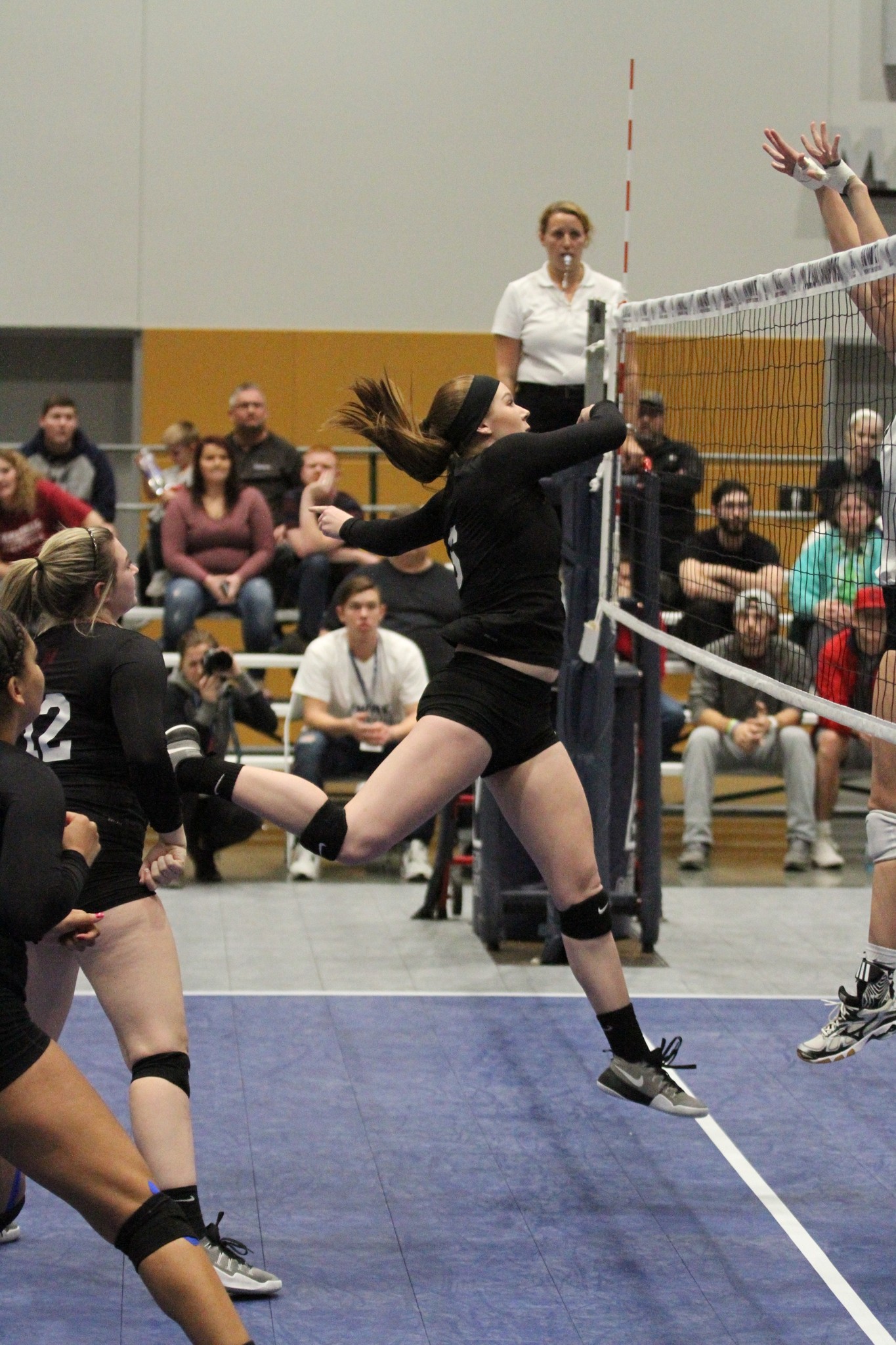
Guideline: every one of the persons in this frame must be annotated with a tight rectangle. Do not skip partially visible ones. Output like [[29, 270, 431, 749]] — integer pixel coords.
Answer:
[[0, 120, 896, 1345]]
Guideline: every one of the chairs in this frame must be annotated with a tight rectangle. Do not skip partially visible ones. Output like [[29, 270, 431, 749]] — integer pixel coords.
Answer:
[[283, 691, 391, 872]]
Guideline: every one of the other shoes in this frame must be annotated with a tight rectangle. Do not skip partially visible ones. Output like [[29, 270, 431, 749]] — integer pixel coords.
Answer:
[[678, 842, 711, 869], [811, 835, 845, 869], [782, 839, 810, 872], [185, 834, 224, 884]]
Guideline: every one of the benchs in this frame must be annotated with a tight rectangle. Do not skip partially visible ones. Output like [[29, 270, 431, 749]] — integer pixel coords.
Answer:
[[0, 441, 871, 820]]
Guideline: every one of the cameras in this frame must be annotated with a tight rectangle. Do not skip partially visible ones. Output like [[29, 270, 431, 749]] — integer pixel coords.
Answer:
[[201, 650, 233, 676]]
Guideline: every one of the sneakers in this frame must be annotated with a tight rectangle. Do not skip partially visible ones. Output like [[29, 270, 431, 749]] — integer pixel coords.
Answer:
[[198, 1210, 283, 1296], [796, 985, 896, 1064], [289, 848, 320, 881], [399, 838, 433, 880], [165, 722, 203, 771], [596, 1036, 709, 1118], [0, 1221, 21, 1242]]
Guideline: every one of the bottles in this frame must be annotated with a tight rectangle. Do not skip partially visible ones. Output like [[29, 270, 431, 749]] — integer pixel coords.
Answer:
[[138, 447, 165, 492]]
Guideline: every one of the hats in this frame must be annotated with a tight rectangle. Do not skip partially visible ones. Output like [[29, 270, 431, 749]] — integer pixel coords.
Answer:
[[734, 588, 777, 620], [637, 396, 664, 418], [712, 480, 754, 509], [854, 587, 886, 611]]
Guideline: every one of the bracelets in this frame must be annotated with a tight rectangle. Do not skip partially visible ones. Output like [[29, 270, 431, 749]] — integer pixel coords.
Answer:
[[725, 718, 738, 734], [730, 721, 741, 737]]
